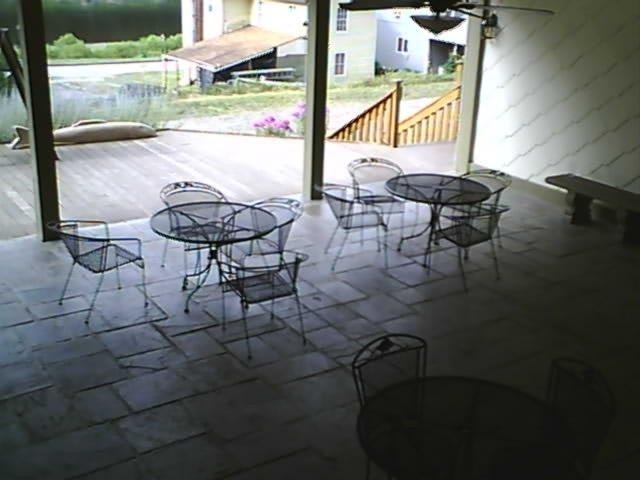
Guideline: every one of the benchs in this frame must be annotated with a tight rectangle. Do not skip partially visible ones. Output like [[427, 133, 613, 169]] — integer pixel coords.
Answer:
[[545, 172, 640, 240]]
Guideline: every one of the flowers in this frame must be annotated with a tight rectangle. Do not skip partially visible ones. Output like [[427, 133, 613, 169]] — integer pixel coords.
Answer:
[[252, 102, 307, 138]]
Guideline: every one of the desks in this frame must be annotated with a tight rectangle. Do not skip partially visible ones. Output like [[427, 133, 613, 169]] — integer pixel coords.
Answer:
[[357, 375, 577, 478]]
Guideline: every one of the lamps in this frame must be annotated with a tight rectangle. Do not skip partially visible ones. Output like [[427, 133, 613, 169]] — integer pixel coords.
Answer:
[[410, 12, 466, 36], [480, 11, 504, 39]]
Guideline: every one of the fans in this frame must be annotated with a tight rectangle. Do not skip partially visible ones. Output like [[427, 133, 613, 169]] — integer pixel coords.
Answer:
[[336, 0, 554, 24]]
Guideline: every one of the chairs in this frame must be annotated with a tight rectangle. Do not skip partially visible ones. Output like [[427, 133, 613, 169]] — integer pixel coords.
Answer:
[[350, 335, 428, 475], [347, 157, 409, 247], [218, 249, 309, 360], [314, 182, 389, 270], [435, 168, 512, 261], [546, 358, 617, 465], [221, 197, 304, 307], [160, 181, 236, 287], [428, 202, 511, 292], [45, 219, 149, 324]]
